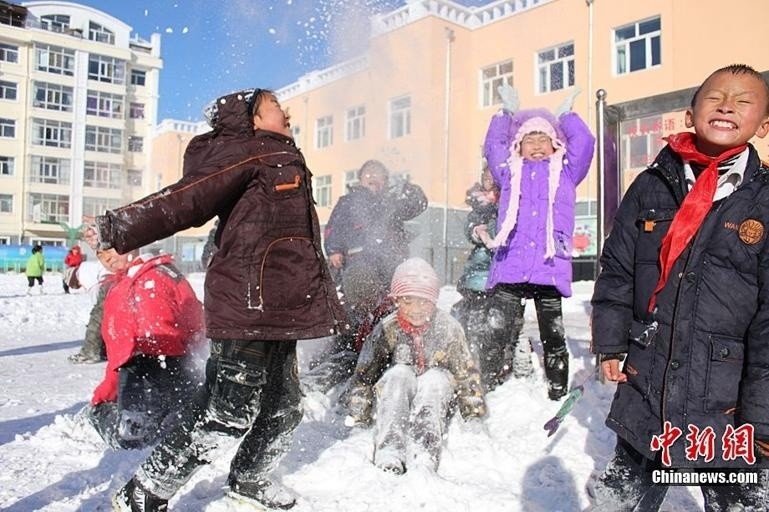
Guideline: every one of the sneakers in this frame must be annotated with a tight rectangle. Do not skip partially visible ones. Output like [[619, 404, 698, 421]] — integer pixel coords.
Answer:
[[112, 475, 168, 512], [225, 472, 296, 510]]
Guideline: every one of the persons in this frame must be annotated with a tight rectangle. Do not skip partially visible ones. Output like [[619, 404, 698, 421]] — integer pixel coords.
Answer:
[[64, 91, 594, 512], [592, 66, 769, 512], [26, 245, 45, 296]]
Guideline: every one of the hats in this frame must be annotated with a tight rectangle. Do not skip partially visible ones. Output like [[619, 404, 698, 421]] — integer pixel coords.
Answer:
[[509, 117, 564, 153], [388, 257, 439, 305]]
